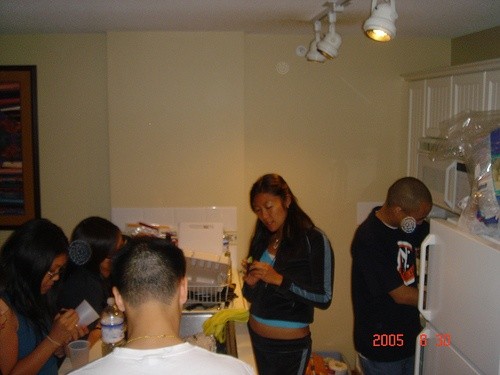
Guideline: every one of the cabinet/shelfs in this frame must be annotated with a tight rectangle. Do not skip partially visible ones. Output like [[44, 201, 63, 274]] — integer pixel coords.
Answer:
[[406, 69, 500, 178]]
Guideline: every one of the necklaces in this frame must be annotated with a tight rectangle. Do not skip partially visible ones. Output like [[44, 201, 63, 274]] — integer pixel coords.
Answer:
[[273, 240, 280, 249]]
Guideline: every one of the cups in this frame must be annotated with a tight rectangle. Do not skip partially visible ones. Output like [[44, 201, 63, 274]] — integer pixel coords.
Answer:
[[67, 340, 89, 370], [73, 299, 100, 326]]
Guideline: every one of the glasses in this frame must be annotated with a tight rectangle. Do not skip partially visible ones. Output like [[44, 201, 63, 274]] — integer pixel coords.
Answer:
[[47, 271, 60, 281]]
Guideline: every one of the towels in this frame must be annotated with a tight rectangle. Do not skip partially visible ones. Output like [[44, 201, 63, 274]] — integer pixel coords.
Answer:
[[200, 307, 251, 344]]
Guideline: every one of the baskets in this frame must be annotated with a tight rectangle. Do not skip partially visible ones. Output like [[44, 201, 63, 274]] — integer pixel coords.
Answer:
[[182, 284, 231, 314]]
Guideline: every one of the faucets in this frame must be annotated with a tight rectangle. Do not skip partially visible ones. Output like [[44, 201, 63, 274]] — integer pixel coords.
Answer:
[[222, 234, 228, 240]]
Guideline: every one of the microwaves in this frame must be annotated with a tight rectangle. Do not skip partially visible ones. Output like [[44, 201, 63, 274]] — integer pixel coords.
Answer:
[[416, 152, 469, 215]]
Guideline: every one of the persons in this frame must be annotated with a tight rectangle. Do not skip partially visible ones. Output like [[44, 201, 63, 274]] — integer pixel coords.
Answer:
[[240, 174, 335, 375], [62, 216, 122, 339], [351, 177, 433, 375], [0, 217, 88, 375], [65, 235, 255, 375]]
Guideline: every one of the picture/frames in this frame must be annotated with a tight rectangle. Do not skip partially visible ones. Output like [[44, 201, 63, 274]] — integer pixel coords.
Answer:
[[0, 64, 42, 231]]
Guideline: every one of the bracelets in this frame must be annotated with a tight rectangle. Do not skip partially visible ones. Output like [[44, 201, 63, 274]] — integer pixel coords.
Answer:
[[47, 336, 60, 345]]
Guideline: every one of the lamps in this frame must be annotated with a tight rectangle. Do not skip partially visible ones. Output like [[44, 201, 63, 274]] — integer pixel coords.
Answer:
[[306, 19, 326, 63], [317, 11, 342, 61], [364, 0, 398, 43]]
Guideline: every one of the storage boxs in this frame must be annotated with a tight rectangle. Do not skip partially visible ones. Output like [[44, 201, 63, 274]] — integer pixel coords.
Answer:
[[311, 351, 352, 375], [178, 222, 231, 295]]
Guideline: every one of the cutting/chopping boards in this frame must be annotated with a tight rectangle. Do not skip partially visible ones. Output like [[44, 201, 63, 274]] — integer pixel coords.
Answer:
[[177, 221, 223, 257]]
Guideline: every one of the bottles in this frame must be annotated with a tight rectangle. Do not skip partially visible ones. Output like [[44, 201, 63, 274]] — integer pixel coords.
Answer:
[[101, 297, 124, 356]]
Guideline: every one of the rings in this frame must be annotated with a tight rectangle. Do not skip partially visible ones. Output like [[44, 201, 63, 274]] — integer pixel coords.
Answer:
[[74, 324, 77, 327]]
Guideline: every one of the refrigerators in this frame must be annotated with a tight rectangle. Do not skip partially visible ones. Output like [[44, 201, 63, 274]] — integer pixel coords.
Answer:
[[412, 217, 500, 375]]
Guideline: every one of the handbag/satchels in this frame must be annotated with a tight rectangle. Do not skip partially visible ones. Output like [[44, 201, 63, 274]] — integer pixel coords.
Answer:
[[305, 352, 331, 375]]
